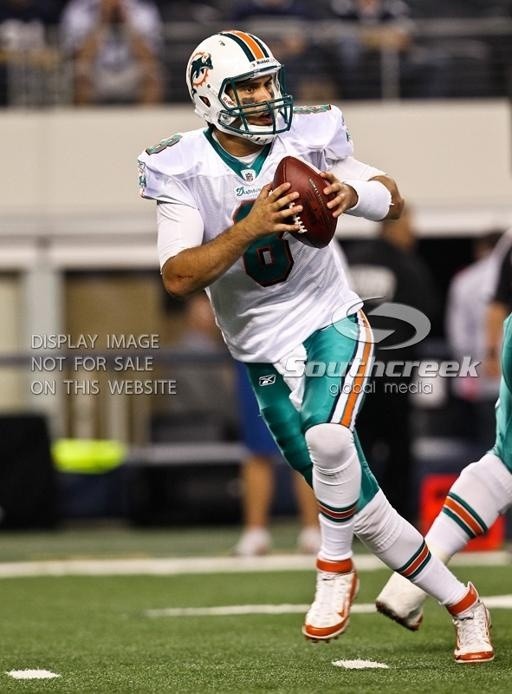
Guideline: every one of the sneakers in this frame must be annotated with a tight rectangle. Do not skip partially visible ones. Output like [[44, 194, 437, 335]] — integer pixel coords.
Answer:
[[374, 574, 424, 630], [448, 582, 496, 664], [301, 559, 360, 645]]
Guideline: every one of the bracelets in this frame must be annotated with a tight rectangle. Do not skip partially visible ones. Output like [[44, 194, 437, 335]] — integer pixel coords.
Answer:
[[339, 177, 394, 223]]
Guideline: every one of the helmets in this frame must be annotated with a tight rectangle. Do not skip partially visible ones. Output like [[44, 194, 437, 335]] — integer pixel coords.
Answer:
[[185, 29, 295, 148]]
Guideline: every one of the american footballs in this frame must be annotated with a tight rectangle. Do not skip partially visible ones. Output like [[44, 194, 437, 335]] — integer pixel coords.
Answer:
[[272, 156, 337, 249]]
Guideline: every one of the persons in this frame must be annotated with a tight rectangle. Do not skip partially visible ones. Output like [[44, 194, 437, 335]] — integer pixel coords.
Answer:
[[346, 199, 446, 529], [229, 359, 321, 560], [3, 1, 512, 107], [136, 26, 498, 665], [371, 311, 512, 634], [448, 234, 507, 460]]
